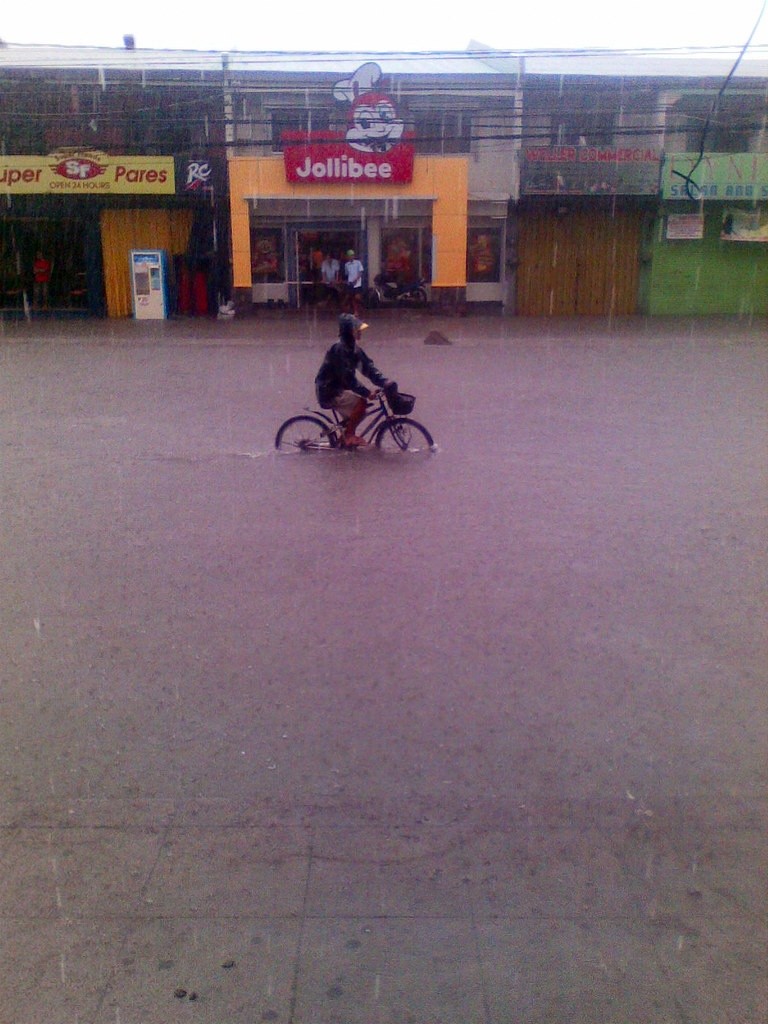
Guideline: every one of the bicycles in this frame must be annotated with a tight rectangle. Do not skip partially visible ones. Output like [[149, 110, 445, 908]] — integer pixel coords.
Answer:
[[275, 383, 434, 452]]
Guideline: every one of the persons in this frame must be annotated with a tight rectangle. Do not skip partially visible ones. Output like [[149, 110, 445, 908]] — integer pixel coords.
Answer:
[[318, 253, 341, 309], [315, 313, 397, 452], [342, 250, 365, 317], [33, 248, 51, 313]]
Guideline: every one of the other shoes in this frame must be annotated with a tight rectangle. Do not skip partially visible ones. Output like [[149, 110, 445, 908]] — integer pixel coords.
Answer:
[[345, 436, 368, 446], [42, 305, 48, 311], [32, 303, 38, 311]]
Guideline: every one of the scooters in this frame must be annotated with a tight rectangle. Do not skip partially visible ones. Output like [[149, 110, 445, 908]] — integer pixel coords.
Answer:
[[370, 269, 427, 307]]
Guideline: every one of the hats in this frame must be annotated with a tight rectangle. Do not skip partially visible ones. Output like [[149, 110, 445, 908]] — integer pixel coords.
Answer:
[[347, 250, 354, 257], [352, 316, 369, 335]]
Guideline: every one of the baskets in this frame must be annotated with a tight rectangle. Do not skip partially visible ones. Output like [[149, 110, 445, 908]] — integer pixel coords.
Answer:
[[386, 392, 416, 415]]
